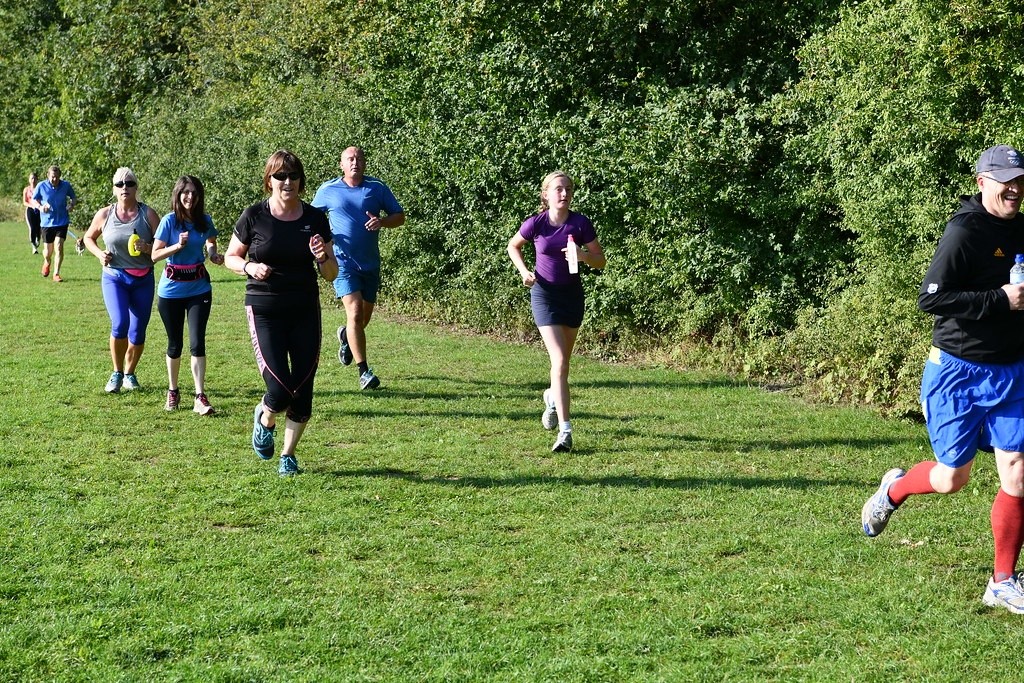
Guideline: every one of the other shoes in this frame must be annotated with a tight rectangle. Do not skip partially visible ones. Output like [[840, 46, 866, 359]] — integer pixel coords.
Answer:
[[32, 246, 38, 254]]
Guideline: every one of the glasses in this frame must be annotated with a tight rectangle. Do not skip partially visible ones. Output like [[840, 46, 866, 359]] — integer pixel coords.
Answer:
[[271, 171, 302, 181], [113, 179, 138, 188], [979, 174, 1024, 189]]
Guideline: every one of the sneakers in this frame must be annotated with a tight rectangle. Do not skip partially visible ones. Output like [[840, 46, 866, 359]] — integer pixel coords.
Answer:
[[52, 274, 64, 282], [42, 260, 51, 277], [543, 388, 559, 429], [861, 467, 907, 538], [358, 368, 381, 390], [165, 387, 181, 412], [122, 374, 140, 389], [336, 325, 353, 365], [252, 403, 278, 459], [982, 572, 1024, 616], [278, 453, 298, 477], [552, 429, 572, 452], [193, 393, 216, 415], [105, 371, 124, 393]]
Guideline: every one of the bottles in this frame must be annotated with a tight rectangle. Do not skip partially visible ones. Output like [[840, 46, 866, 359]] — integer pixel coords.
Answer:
[[1010, 255, 1024, 287], [567, 235, 578, 274], [128, 229, 141, 256]]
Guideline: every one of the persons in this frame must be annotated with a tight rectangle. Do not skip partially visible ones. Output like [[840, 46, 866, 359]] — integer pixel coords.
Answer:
[[860, 145, 1024, 615], [506, 172, 606, 452], [309, 146, 405, 390], [83, 166, 161, 393], [224, 149, 338, 478], [22, 163, 78, 282], [151, 174, 224, 416]]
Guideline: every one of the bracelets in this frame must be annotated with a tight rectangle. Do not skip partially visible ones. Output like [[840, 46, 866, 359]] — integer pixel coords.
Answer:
[[243, 261, 253, 276], [316, 253, 329, 264]]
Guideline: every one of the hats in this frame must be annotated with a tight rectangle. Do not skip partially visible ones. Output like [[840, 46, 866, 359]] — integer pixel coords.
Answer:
[[976, 145, 1024, 183]]
